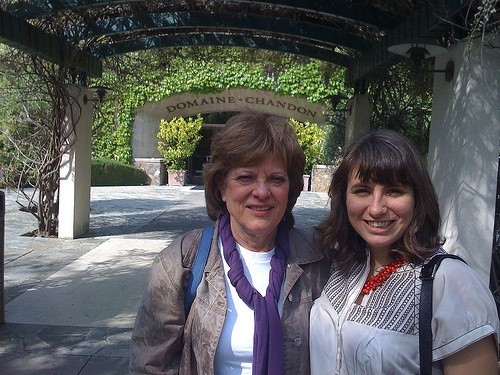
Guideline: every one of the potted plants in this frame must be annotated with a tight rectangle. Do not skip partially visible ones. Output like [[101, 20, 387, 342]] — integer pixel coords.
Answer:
[[156, 114, 203, 187], [290, 117, 330, 191]]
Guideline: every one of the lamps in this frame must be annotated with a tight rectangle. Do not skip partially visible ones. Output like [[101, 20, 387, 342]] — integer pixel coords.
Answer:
[[82, 86, 116, 105], [387, 38, 454, 82]]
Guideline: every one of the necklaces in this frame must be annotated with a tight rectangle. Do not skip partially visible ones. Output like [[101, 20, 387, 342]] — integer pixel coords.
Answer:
[[359, 255, 417, 296]]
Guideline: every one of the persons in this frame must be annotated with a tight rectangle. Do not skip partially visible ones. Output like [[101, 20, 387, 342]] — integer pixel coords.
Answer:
[[128, 110, 332, 375], [307, 124, 500, 375]]
